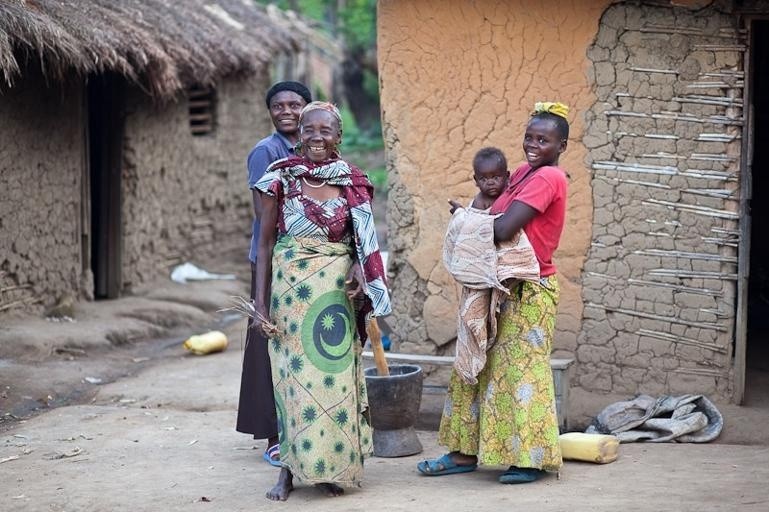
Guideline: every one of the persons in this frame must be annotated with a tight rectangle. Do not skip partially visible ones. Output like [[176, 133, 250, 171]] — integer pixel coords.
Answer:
[[417, 102, 568, 483], [472, 148, 511, 209], [252, 100, 395, 501], [236, 82, 312, 466]]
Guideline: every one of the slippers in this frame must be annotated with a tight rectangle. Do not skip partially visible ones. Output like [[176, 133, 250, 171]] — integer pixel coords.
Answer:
[[263, 444, 282, 467], [499, 467, 537, 484], [417, 454, 474, 476]]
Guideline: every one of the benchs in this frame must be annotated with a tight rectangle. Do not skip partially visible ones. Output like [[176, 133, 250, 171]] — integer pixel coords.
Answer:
[[362, 350, 575, 435]]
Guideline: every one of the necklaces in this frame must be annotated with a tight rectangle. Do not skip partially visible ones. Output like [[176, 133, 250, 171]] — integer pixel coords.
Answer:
[[302, 177, 329, 188]]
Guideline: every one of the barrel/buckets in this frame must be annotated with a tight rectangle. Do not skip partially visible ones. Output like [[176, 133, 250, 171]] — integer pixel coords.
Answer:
[[183, 331, 228, 355]]
